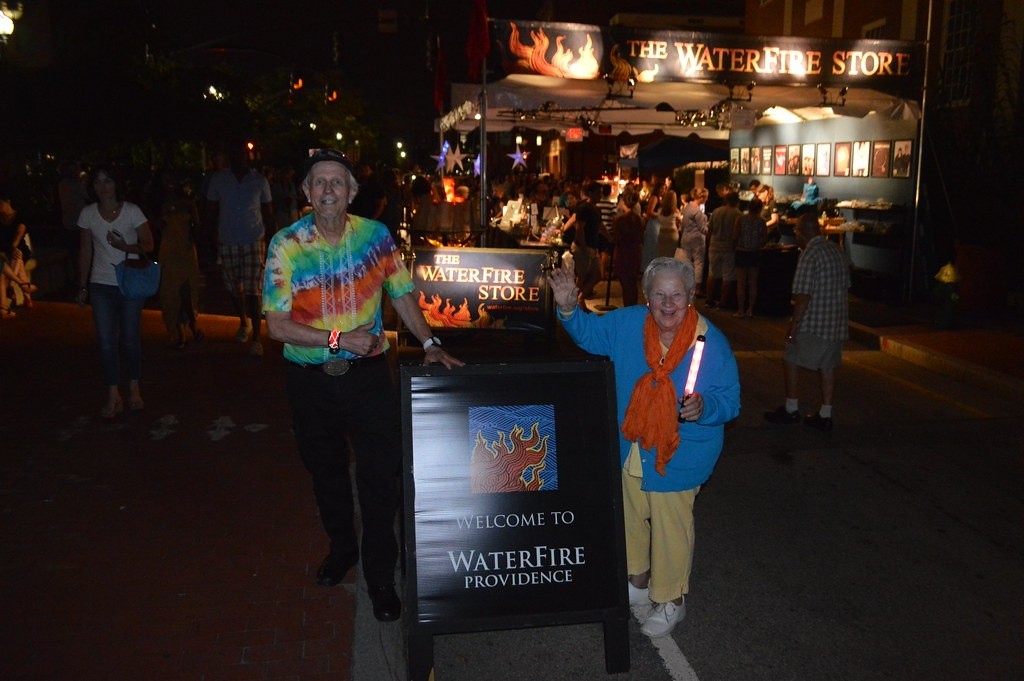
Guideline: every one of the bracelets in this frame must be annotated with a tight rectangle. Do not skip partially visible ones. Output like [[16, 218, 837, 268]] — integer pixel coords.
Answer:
[[558, 308, 574, 316], [328, 330, 341, 355]]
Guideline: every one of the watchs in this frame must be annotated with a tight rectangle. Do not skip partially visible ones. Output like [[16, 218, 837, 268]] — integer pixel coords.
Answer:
[[79, 287, 88, 292], [423, 336, 442, 352]]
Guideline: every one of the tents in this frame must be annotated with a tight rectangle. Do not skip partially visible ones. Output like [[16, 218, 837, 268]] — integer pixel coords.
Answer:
[[429, 15, 935, 248]]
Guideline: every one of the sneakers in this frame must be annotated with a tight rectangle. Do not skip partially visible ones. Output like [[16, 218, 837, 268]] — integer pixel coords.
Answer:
[[628, 581, 650, 605], [641, 594, 685, 637]]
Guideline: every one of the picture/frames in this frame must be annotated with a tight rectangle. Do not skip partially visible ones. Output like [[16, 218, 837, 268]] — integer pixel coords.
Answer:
[[730, 138, 914, 180]]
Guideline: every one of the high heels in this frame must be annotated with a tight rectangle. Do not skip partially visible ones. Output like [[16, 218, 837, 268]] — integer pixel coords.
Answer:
[[131, 398, 144, 410], [101, 400, 123, 419]]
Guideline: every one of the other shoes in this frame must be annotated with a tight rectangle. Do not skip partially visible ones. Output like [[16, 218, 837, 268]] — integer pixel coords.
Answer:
[[704, 301, 716, 307], [746, 312, 753, 319], [716, 303, 732, 310], [733, 311, 744, 318], [248, 342, 262, 356], [235, 329, 250, 343]]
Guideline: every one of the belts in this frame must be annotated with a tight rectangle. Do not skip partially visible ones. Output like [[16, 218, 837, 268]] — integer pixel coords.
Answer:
[[305, 355, 373, 376]]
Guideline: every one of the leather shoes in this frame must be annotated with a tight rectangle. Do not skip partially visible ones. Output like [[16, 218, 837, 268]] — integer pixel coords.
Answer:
[[764, 405, 801, 424], [369, 582, 399, 621], [316, 546, 359, 587], [805, 411, 833, 431]]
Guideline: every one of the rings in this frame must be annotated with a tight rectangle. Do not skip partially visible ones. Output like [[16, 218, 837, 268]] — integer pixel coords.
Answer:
[[788, 336, 792, 339], [108, 240, 112, 244]]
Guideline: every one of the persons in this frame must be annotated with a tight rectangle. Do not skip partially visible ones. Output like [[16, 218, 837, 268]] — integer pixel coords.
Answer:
[[146, 163, 209, 349], [487, 169, 779, 319], [75, 161, 156, 418], [350, 149, 481, 249], [791, 177, 823, 211], [258, 146, 467, 621], [196, 133, 305, 356], [762, 211, 851, 432], [0, 186, 38, 319], [541, 258, 740, 640]]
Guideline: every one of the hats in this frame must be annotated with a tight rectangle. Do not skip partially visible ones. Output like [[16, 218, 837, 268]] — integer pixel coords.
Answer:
[[303, 149, 352, 177]]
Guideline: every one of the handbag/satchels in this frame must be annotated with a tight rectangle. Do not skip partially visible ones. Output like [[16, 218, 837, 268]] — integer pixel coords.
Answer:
[[114, 244, 163, 299]]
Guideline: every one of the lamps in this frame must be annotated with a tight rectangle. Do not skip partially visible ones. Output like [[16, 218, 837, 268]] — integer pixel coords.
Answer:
[[722, 78, 757, 102], [601, 74, 636, 99], [815, 83, 849, 108]]
